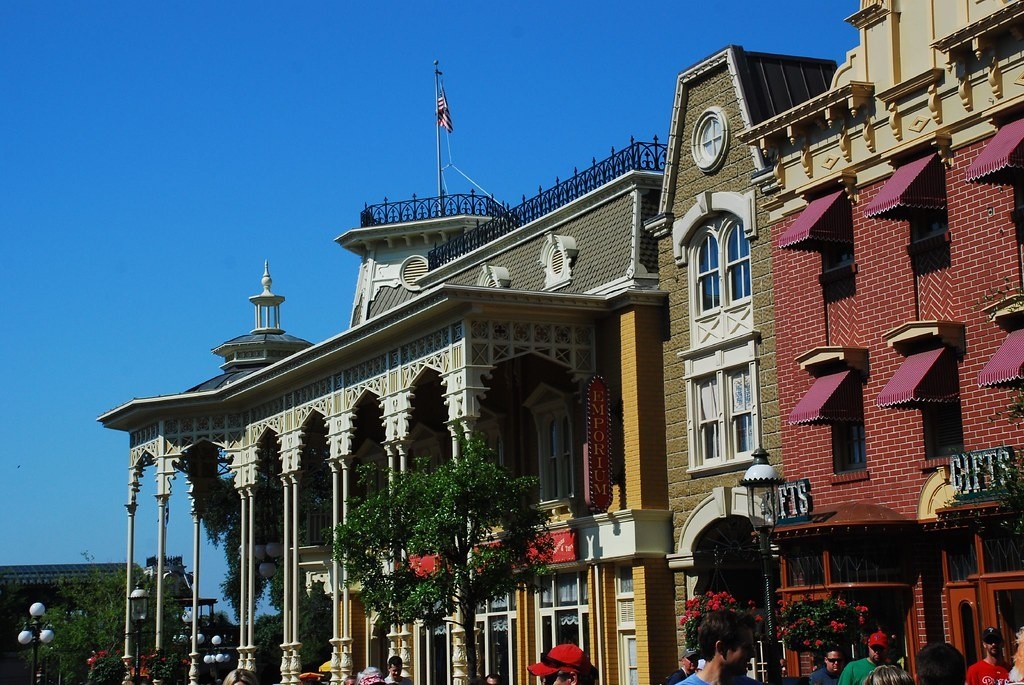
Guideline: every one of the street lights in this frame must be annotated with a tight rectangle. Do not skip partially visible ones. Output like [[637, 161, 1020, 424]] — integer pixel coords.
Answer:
[[18, 602, 54, 685], [173, 610, 205, 685], [128, 579, 151, 685], [203, 635, 231, 685], [739, 443, 787, 685]]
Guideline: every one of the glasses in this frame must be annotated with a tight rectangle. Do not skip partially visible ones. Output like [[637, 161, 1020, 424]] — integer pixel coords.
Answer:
[[872, 645, 884, 652], [828, 658, 841, 663], [985, 638, 1001, 644]]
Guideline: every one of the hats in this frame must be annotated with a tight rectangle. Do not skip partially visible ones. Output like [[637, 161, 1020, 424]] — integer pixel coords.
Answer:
[[682, 648, 700, 658], [528, 644, 587, 677], [982, 627, 1002, 641], [300, 672, 324, 682], [358, 667, 385, 685], [868, 630, 889, 648]]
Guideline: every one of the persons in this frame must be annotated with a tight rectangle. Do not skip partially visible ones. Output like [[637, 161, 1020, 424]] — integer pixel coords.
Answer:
[[221, 609, 1024, 685]]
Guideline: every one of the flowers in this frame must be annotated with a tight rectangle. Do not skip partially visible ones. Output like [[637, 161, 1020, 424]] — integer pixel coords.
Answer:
[[680, 589, 766, 652], [774, 595, 872, 651]]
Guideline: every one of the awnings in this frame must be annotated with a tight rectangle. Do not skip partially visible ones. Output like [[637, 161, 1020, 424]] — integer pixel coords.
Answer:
[[789, 370, 864, 424], [875, 347, 960, 408], [777, 190, 854, 254], [966, 118, 1024, 185], [862, 154, 946, 221], [976, 328, 1024, 390]]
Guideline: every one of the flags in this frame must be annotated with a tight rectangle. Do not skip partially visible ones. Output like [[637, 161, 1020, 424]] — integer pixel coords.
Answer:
[[435, 85, 453, 133]]
